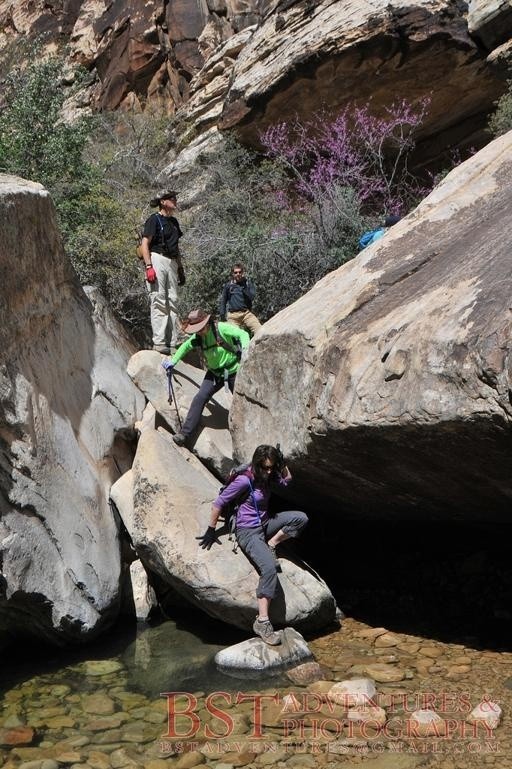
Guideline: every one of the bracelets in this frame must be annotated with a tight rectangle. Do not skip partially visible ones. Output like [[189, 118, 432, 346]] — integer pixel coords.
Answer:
[[145, 263, 152, 266]]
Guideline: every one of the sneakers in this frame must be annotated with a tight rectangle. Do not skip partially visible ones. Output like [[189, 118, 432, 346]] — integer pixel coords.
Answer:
[[266, 541, 281, 570], [152, 343, 171, 356], [252, 614, 282, 646], [172, 432, 187, 447]]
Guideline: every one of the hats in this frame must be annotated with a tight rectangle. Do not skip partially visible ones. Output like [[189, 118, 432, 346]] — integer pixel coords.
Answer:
[[148, 189, 182, 208], [184, 309, 212, 334]]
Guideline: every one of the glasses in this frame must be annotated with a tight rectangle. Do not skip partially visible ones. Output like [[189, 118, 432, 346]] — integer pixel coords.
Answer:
[[259, 463, 276, 471], [233, 271, 242, 274], [165, 196, 178, 202]]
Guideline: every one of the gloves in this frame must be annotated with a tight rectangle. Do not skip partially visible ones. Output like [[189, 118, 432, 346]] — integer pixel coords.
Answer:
[[220, 314, 228, 322], [195, 525, 223, 551], [144, 263, 158, 284], [177, 266, 186, 287], [161, 359, 175, 370]]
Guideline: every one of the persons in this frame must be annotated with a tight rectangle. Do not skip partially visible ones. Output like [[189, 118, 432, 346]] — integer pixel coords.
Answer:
[[160, 308, 251, 447], [219, 263, 262, 335], [358, 215, 401, 248], [138, 188, 187, 355], [197, 444, 309, 644]]
